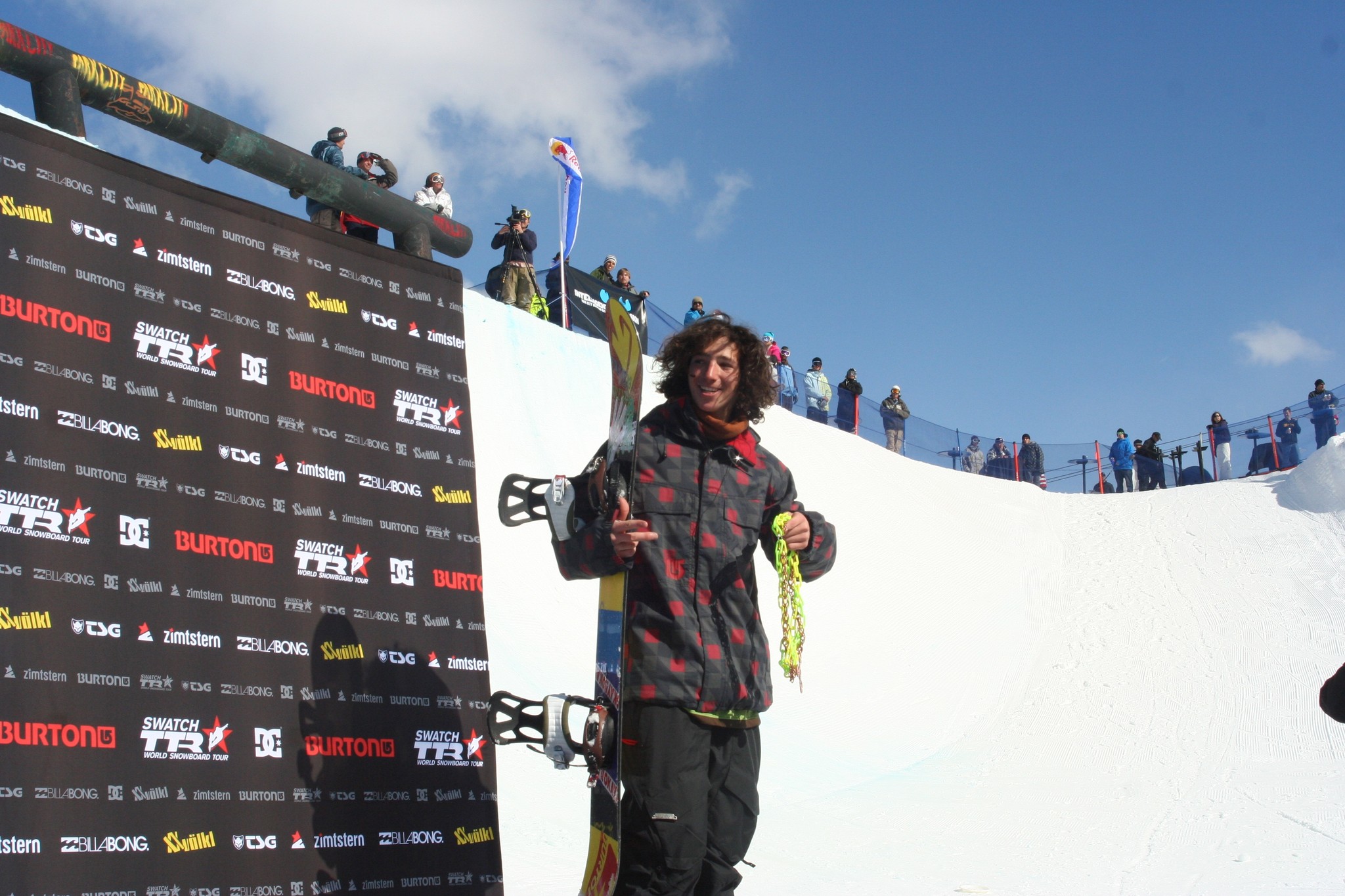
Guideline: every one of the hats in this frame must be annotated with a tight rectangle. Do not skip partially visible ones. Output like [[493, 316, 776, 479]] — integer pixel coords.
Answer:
[[692, 297, 703, 308], [812, 357, 822, 367], [327, 127, 374, 165], [848, 368, 856, 378], [763, 332, 774, 342], [1116, 428, 1162, 446], [603, 255, 617, 268], [891, 385, 900, 391], [1314, 379, 1325, 388], [551, 251, 569, 261]]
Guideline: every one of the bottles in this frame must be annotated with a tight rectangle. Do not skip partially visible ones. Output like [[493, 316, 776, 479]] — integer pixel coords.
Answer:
[[1288, 427, 1291, 434]]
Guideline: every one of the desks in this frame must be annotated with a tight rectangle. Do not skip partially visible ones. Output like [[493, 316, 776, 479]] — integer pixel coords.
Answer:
[[988, 458, 1031, 482], [1306, 408, 1342, 438], [1067, 459, 1098, 494], [1248, 442, 1282, 472], [937, 450, 971, 470], [1237, 433, 1271, 474]]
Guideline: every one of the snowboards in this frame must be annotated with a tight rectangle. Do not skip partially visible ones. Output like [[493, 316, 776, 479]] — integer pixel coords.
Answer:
[[577, 296, 643, 896]]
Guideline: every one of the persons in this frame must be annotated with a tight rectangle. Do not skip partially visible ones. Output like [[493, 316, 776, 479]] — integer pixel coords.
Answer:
[[1308, 379, 1339, 450], [987, 438, 1011, 464], [1206, 412, 1232, 481], [962, 436, 984, 475], [880, 385, 910, 454], [684, 297, 706, 329], [1276, 407, 1301, 468], [343, 151, 398, 243], [1018, 434, 1045, 487], [1141, 432, 1167, 491], [306, 127, 370, 234], [1108, 428, 1134, 493], [1132, 439, 1150, 492], [834, 368, 863, 434], [486, 209, 569, 327], [550, 312, 836, 896], [1093, 472, 1115, 493], [590, 255, 617, 285], [804, 357, 832, 425], [412, 173, 453, 219], [761, 332, 782, 382], [614, 268, 650, 297], [776, 346, 799, 412]]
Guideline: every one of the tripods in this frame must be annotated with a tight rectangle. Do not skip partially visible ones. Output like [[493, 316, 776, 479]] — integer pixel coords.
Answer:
[[495, 226, 549, 322]]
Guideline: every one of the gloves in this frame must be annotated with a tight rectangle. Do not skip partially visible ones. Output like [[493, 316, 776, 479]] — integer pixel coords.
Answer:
[[793, 396, 827, 408], [371, 152, 382, 163], [1206, 424, 1213, 429]]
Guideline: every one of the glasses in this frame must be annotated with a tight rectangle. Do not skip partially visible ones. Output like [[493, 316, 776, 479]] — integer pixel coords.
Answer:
[[328, 129, 444, 184], [813, 361, 821, 366], [847, 371, 856, 376], [519, 209, 531, 219], [1117, 432, 1124, 434], [693, 302, 701, 305], [971, 439, 980, 443], [780, 350, 790, 357], [762, 336, 773, 342], [1319, 383, 1324, 385], [891, 389, 900, 393]]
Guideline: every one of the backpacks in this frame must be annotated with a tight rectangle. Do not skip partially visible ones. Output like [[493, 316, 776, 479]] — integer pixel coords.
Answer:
[[530, 293, 548, 322]]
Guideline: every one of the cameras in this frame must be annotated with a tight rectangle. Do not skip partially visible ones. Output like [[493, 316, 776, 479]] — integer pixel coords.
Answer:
[[508, 206, 523, 227]]
